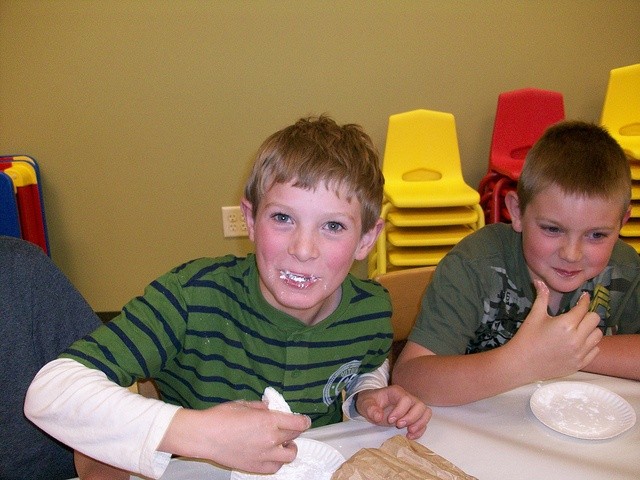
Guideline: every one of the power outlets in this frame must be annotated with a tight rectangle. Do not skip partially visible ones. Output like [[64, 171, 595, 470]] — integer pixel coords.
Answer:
[[221, 205, 249, 239]]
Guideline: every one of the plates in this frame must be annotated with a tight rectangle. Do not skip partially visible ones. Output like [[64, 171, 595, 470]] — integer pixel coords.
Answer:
[[230, 436, 346, 480], [530, 381, 636, 440]]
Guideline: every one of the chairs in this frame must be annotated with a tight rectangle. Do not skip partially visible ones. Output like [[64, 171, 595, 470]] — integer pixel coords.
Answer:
[[366, 109, 486, 278], [597, 63, 640, 255], [479, 87, 566, 226]]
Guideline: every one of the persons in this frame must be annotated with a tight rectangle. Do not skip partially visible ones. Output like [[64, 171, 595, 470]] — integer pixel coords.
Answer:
[[0, 236, 130, 480], [24, 114, 432, 480], [392, 121, 640, 407]]
